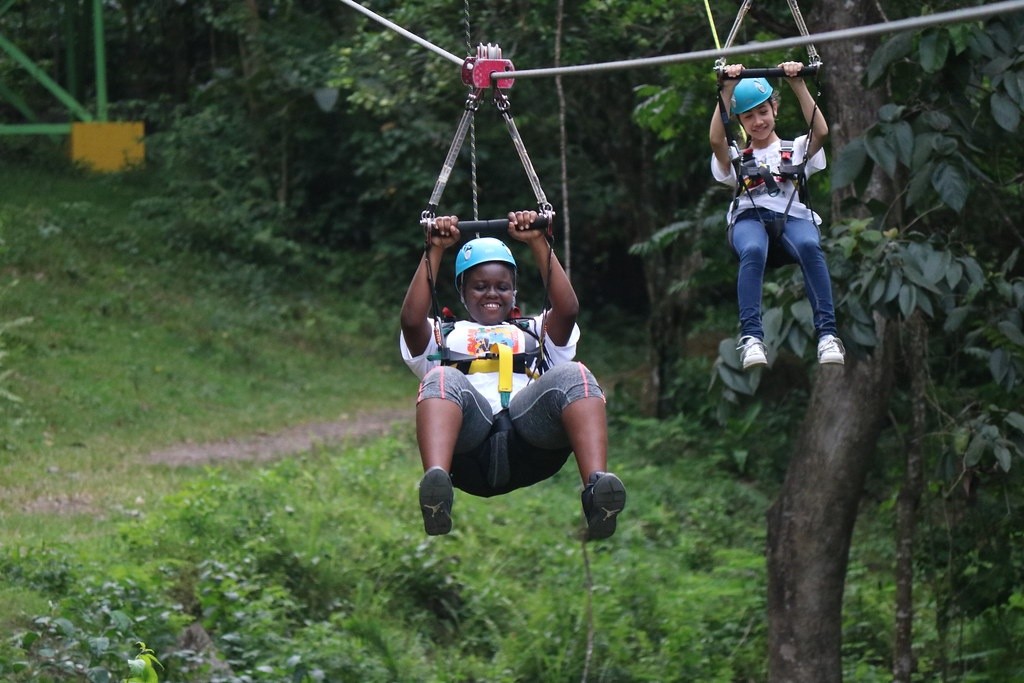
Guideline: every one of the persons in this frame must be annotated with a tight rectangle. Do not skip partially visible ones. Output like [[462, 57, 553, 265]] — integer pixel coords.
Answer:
[[399, 210, 627, 539], [708, 60, 847, 370]]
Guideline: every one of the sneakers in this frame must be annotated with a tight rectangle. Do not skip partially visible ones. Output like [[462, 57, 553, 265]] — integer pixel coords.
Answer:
[[419, 467, 453, 535], [817, 335, 846, 365], [737, 336, 769, 369], [582, 469, 626, 539]]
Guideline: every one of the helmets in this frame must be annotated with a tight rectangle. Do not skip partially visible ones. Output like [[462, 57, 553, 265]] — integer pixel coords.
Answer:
[[731, 78, 773, 114], [454, 237, 516, 292]]
